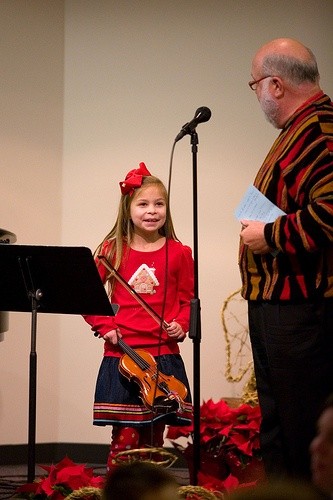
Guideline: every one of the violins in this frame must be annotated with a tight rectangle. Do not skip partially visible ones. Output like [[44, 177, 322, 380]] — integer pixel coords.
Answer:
[[94, 330, 188, 413]]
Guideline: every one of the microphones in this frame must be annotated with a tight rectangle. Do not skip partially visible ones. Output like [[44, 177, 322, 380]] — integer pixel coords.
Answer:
[[175, 106, 211, 141]]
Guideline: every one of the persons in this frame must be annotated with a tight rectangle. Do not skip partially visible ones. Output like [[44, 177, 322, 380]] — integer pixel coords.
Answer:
[[310, 404, 333, 500], [227, 475, 323, 500], [239, 38, 333, 484], [79, 175, 194, 477], [103, 461, 182, 500]]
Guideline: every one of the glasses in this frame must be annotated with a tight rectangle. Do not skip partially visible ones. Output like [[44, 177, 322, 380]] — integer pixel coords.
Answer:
[[248, 76, 274, 90]]
